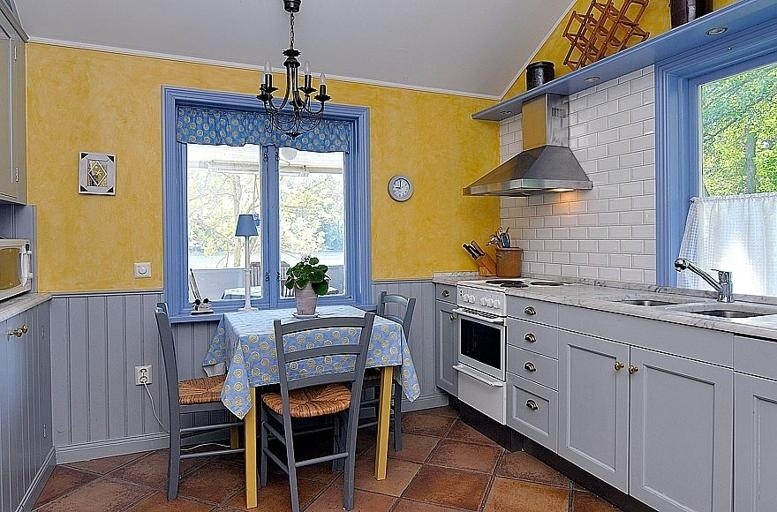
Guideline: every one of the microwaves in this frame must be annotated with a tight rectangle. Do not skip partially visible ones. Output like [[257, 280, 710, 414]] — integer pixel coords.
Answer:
[[0, 239, 34, 301]]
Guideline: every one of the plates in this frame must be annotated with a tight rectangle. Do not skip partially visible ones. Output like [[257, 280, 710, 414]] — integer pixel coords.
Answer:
[[292, 312, 318, 319]]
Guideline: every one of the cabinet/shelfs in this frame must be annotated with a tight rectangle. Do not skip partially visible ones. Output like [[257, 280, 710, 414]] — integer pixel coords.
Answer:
[[506, 295, 559, 454], [734, 332, 776, 512], [558, 310, 733, 512], [1, 300, 52, 512], [0, 9, 27, 205], [434, 285, 457, 395]]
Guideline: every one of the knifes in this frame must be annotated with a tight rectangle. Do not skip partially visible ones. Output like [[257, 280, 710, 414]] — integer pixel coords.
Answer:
[[462, 240, 484, 259]]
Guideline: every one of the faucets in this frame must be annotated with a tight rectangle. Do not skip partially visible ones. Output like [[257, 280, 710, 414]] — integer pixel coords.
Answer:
[[674, 258, 734, 303]]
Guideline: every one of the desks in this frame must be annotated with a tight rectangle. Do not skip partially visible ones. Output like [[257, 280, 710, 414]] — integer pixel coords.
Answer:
[[201, 303, 421, 508], [221, 286, 339, 299]]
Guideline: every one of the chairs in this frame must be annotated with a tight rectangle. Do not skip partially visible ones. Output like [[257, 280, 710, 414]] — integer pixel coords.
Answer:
[[259, 311, 376, 512], [153, 301, 245, 503], [280, 260, 290, 280], [281, 279, 297, 297], [250, 261, 261, 287], [337, 289, 417, 468], [189, 269, 202, 300]]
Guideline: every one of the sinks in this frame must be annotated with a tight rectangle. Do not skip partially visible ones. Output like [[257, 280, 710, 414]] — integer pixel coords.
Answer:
[[592, 292, 707, 307], [664, 302, 777, 320]]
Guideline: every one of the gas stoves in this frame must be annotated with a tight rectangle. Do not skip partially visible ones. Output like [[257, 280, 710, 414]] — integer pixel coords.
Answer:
[[458, 276, 574, 288]]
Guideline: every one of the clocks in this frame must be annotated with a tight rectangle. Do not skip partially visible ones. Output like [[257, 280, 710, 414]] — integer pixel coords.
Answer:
[[389, 175, 414, 202]]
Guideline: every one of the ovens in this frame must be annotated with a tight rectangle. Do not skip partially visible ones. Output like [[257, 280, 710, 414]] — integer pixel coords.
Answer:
[[452, 285, 506, 426]]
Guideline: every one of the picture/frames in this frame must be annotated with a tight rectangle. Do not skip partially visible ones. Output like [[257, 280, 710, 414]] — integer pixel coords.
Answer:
[[78, 151, 116, 196]]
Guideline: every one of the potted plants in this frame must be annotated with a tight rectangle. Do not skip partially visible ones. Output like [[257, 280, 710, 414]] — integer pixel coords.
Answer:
[[283, 252, 332, 315]]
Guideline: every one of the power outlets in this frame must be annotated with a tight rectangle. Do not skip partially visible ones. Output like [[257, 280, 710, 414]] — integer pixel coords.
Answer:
[[135, 365, 153, 385]]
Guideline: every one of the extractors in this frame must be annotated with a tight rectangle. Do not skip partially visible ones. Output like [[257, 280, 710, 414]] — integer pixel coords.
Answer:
[[463, 94, 593, 197]]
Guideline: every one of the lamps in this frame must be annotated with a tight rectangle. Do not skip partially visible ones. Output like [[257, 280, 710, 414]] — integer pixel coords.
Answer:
[[235, 213, 259, 311], [254, 0, 331, 141]]
